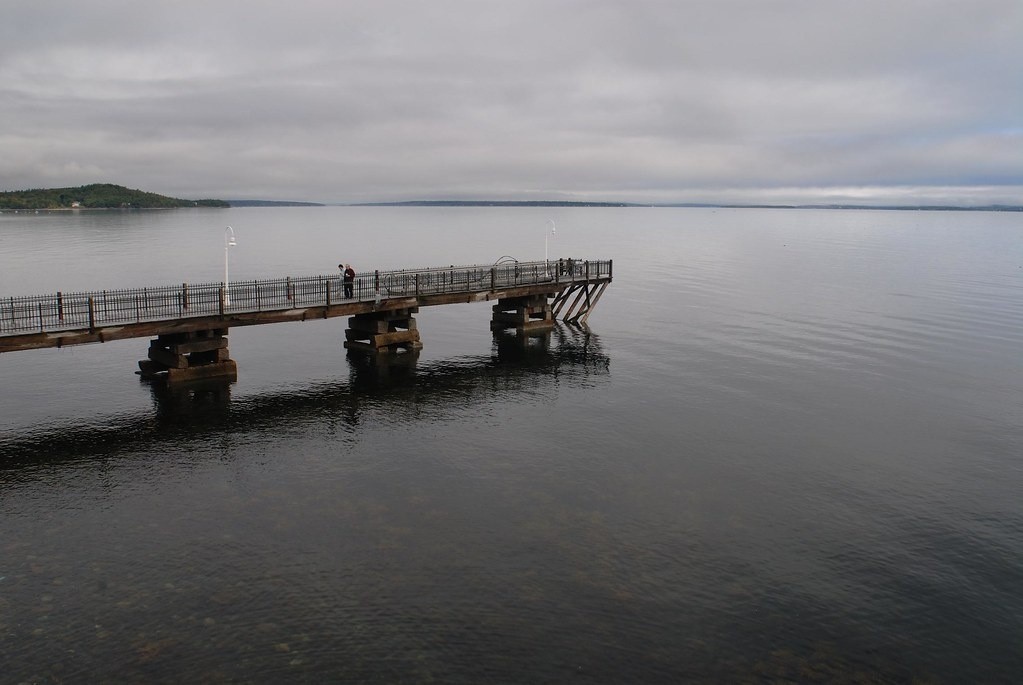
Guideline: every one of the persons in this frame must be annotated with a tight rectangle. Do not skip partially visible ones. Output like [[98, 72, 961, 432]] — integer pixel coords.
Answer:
[[337, 264, 346, 298], [344, 263, 356, 298]]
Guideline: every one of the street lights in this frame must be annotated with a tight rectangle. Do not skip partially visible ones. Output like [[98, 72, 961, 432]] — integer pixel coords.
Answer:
[[544, 218, 557, 278], [223, 224, 236, 308]]
